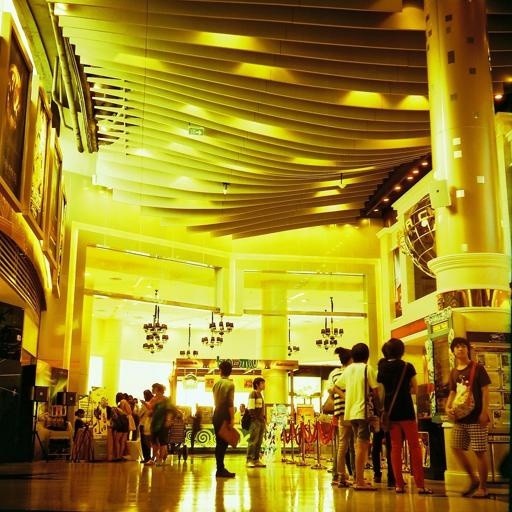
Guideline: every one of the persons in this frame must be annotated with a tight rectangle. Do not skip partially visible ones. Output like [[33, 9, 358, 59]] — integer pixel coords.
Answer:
[[69, 381, 174, 466], [211, 360, 236, 478], [376, 337, 433, 495], [325, 342, 396, 492], [502, 355, 509, 366], [243, 377, 266, 468], [443, 337, 493, 499], [187, 402, 252, 453]]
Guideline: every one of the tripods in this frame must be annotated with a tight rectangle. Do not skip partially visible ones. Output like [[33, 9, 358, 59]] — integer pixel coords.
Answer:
[[31, 402, 49, 463]]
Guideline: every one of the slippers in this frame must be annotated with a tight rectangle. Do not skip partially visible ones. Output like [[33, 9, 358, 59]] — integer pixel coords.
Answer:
[[417, 487, 432, 493]]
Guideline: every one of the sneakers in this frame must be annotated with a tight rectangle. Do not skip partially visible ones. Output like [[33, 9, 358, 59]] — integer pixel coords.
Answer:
[[332, 475, 407, 493], [217, 470, 235, 477], [245, 460, 266, 467]]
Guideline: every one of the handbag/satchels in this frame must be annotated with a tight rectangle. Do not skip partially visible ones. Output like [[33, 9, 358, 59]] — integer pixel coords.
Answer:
[[451, 389, 475, 419], [322, 394, 334, 414], [219, 421, 240, 447], [242, 408, 256, 436], [381, 412, 390, 431], [369, 416, 380, 432]]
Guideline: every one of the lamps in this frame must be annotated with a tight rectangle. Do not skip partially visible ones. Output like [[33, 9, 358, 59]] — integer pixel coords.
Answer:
[[315, 297, 345, 351], [201, 312, 234, 349], [142, 289, 169, 354]]
